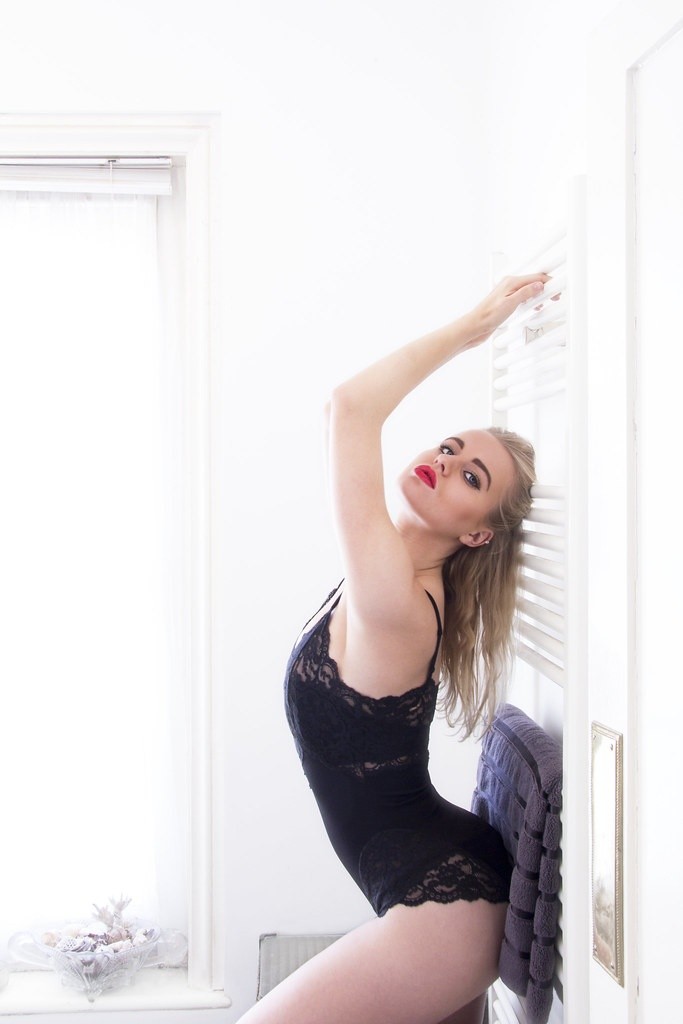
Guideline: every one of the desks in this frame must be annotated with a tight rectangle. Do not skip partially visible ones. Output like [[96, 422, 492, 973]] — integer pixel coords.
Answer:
[[1, 966, 231, 1015]]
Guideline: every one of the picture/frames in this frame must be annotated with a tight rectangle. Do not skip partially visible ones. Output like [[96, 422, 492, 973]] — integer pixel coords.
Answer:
[[589, 720, 624, 988]]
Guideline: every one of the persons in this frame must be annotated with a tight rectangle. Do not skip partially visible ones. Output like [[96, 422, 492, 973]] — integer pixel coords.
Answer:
[[233, 272, 560, 1024]]
